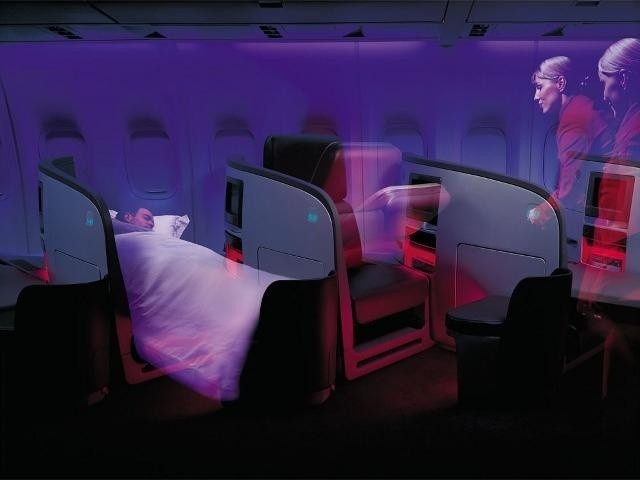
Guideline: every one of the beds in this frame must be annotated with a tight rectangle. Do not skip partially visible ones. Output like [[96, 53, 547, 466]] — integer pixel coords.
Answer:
[[398, 149, 569, 356], [37, 155, 335, 427], [224, 155, 439, 379], [557, 149, 639, 309]]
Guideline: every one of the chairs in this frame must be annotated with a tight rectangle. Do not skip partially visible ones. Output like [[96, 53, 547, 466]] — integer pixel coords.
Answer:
[[2, 274, 107, 443]]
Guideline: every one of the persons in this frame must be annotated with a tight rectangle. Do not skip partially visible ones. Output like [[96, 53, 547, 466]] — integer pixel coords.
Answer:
[[527, 52, 616, 244], [580, 39, 640, 408], [112, 203, 155, 234]]
